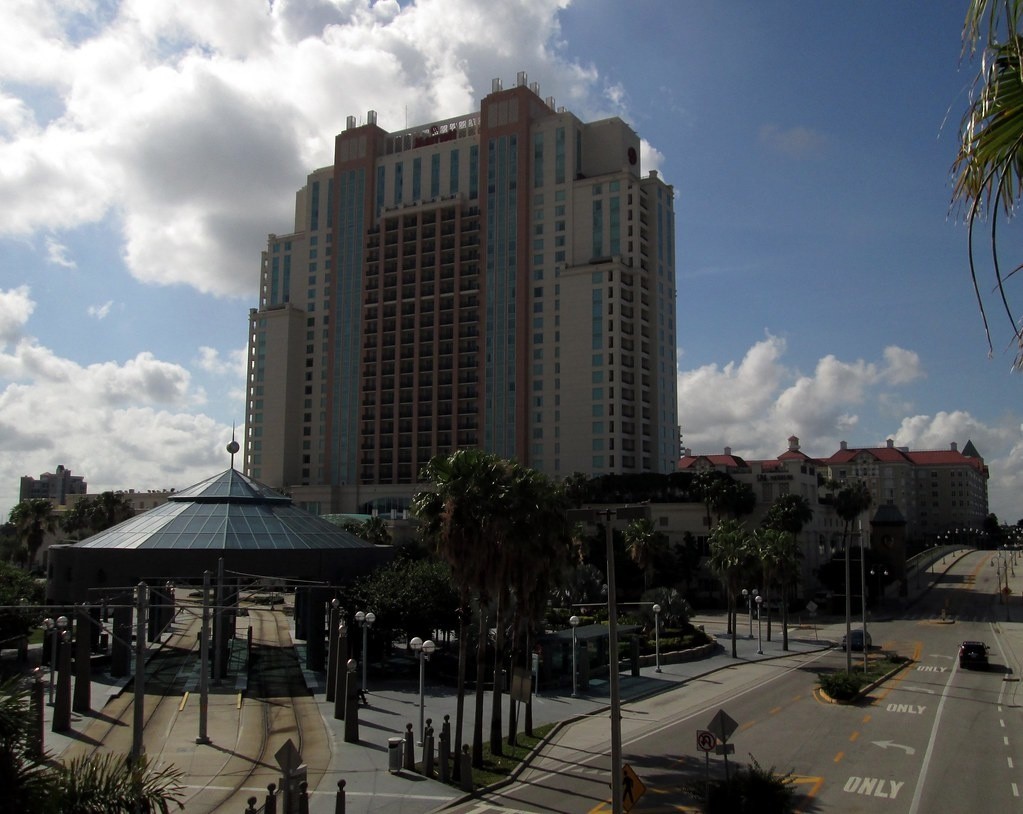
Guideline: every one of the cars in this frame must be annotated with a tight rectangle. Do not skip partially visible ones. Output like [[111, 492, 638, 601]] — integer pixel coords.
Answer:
[[841, 631, 872, 650]]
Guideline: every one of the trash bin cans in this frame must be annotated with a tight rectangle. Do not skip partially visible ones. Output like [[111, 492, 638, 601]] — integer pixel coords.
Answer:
[[388, 736, 403, 774]]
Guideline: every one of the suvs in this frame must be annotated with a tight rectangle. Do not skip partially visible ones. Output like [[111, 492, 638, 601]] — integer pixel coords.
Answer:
[[958, 641, 991, 670]]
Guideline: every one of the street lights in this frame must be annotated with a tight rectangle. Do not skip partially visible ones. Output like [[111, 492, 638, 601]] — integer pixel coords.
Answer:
[[652, 604, 661, 672], [41, 615, 67, 705], [869, 562, 889, 616], [990, 528, 1023, 621], [570, 616, 580, 699], [755, 596, 763, 654], [409, 637, 435, 747], [572, 504, 653, 814], [915, 527, 988, 590], [741, 588, 759, 639], [354, 611, 375, 693]]
